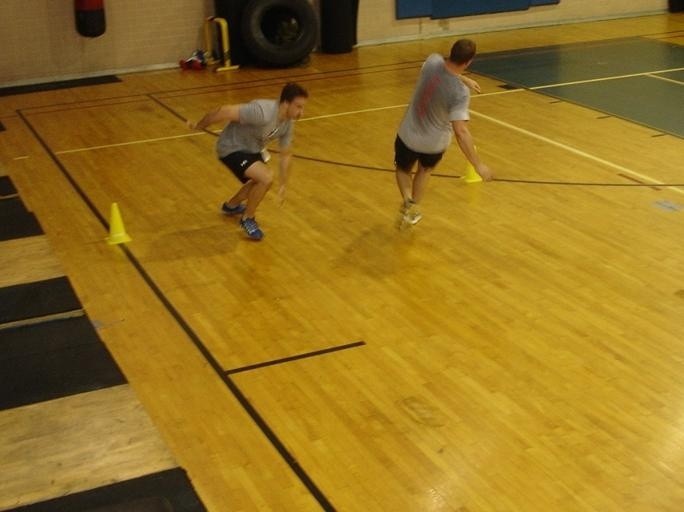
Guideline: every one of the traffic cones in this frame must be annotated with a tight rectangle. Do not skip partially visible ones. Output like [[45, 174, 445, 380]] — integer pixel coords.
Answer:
[[462, 145, 483, 183], [104, 201, 132, 245]]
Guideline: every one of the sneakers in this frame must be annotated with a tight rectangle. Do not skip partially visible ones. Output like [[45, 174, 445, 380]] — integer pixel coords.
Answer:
[[222, 202, 246, 215], [409, 212, 422, 224], [240, 217, 262, 239]]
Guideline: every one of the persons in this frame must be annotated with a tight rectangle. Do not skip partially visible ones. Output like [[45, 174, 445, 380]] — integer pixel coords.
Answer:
[[187, 82, 309, 240], [395, 38, 494, 224]]
